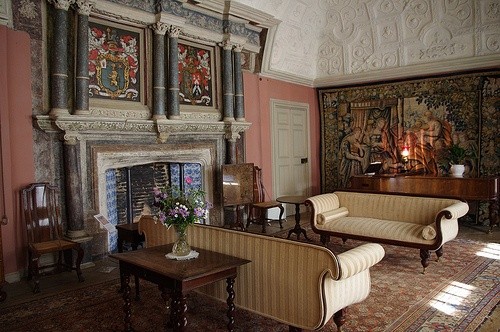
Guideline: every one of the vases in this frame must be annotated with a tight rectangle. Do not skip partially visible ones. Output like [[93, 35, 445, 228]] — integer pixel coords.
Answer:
[[172, 231, 191, 256]]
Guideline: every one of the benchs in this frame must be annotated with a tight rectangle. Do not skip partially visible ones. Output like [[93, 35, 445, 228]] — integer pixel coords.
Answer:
[[305, 189, 470, 274], [137, 214, 385, 331]]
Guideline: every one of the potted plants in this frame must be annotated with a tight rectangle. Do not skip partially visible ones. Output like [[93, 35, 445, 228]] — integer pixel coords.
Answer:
[[441, 147, 470, 177]]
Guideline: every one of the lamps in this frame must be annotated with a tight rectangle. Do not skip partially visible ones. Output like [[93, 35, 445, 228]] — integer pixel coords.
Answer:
[[401, 146, 409, 170]]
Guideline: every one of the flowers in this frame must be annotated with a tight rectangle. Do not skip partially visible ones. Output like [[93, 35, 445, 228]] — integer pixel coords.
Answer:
[[153, 177, 211, 230]]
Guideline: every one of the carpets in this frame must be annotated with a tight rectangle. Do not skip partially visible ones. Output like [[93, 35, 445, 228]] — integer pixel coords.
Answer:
[[0, 230, 500, 332]]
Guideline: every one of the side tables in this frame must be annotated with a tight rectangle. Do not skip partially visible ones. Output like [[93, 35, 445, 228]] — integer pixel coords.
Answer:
[[278, 196, 313, 242]]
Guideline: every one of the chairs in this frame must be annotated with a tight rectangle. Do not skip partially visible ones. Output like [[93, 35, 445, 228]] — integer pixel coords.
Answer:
[[20, 183, 86, 293], [247, 165, 284, 231]]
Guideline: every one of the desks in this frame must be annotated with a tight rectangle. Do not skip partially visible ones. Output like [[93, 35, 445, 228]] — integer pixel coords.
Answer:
[[109, 244, 249, 332], [351, 175, 500, 229]]
